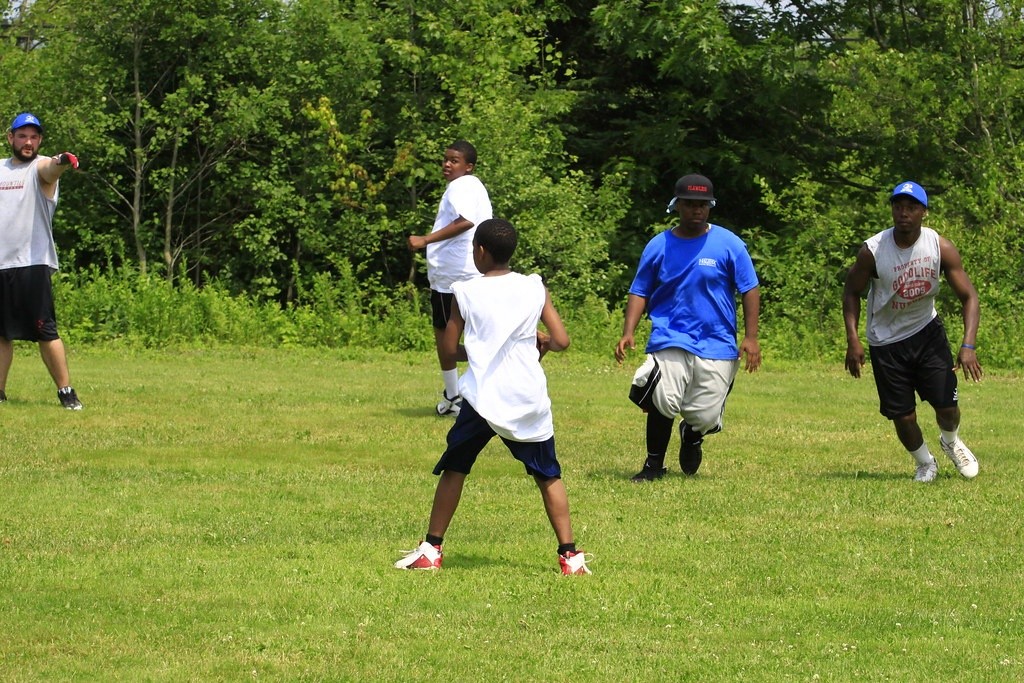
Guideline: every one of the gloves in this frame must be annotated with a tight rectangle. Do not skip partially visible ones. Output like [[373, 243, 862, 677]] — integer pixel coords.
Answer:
[[55, 151, 80, 169]]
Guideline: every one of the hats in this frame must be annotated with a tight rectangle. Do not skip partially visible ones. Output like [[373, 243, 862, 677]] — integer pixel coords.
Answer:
[[889, 181, 928, 207], [9, 113, 43, 133], [675, 173, 717, 202]]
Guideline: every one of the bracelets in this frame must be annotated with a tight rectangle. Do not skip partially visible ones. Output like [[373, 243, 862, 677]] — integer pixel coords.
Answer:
[[961, 344, 976, 350]]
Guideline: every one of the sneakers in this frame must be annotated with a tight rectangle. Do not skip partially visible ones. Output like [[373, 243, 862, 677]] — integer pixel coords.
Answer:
[[559, 551, 592, 574], [393, 540, 442, 570], [940, 436, 979, 479], [630, 458, 667, 481], [57, 386, 82, 410], [914, 457, 939, 482], [436, 389, 461, 416], [678, 418, 704, 476], [0, 390, 8, 402]]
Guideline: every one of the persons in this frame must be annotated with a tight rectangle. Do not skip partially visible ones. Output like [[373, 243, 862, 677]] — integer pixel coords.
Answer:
[[393, 218, 594, 577], [612, 171, 762, 485], [843, 181, 980, 482], [407, 140, 495, 418], [0, 113, 82, 412]]
